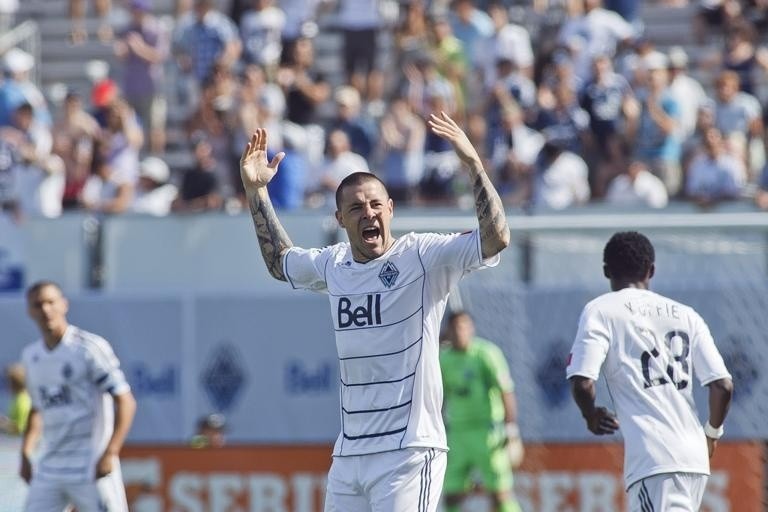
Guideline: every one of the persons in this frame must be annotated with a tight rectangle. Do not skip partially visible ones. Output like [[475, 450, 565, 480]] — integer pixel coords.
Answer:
[[564, 232, 736, 509], [189, 413, 229, 445], [439, 310, 524, 509], [1, 358, 34, 435], [0, 0, 768, 223], [241, 110, 513, 509], [18, 279, 136, 510]]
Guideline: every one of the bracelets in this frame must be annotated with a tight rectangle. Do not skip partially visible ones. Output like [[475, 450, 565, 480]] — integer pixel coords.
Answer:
[[704, 417, 730, 440]]
